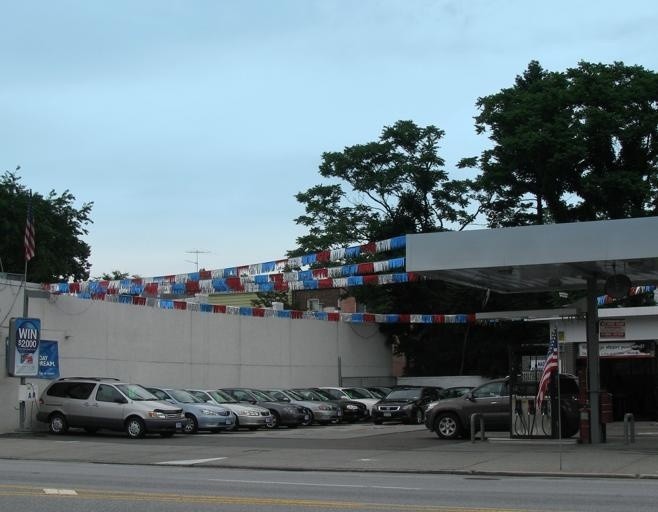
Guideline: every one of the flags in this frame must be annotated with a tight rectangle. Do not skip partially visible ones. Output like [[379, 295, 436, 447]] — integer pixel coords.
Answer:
[[536, 329, 558, 411]]
